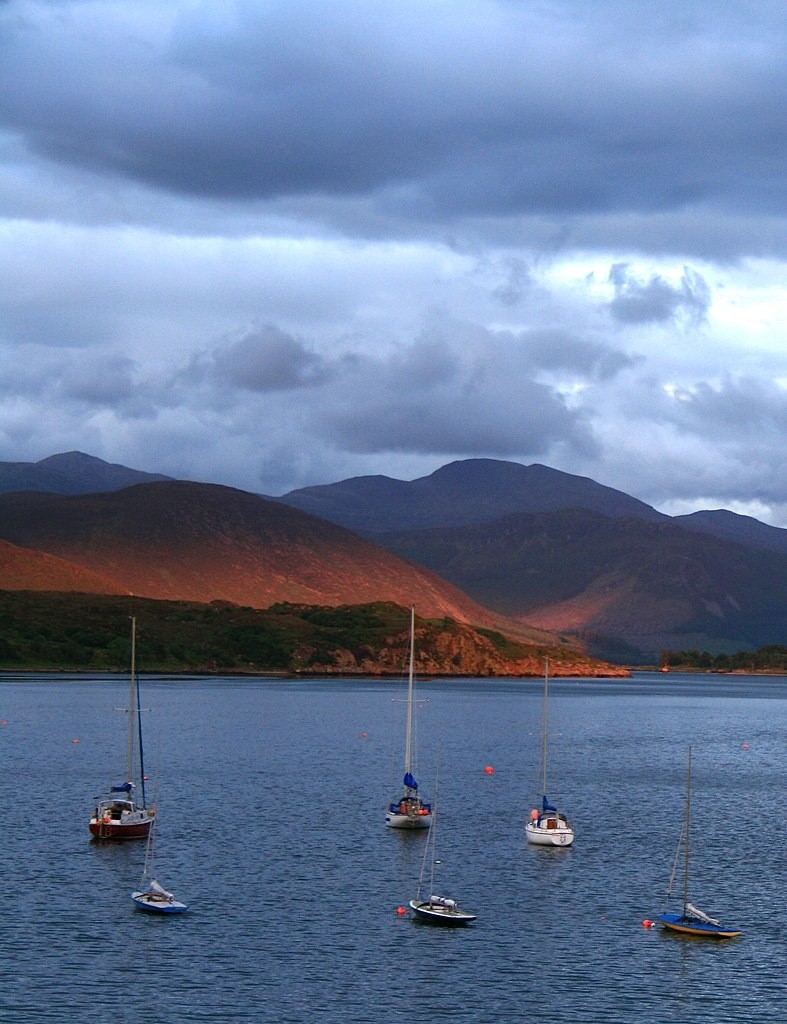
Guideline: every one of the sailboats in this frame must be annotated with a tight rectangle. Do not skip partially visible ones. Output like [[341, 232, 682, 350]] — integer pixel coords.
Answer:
[[88, 614, 155, 840], [658, 745, 744, 937], [409, 760, 479, 922], [130, 788, 191, 914], [384, 604, 436, 829], [525, 653, 575, 847]]
